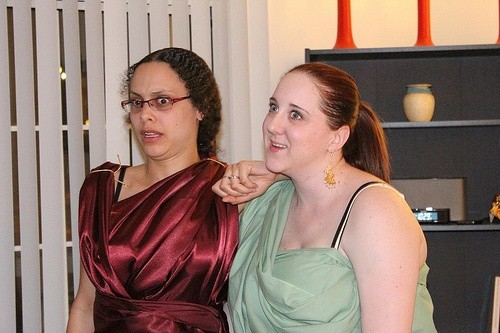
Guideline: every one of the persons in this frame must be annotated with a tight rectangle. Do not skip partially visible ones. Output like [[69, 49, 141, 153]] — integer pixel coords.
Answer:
[[65, 48, 290, 333], [223, 63, 437, 333]]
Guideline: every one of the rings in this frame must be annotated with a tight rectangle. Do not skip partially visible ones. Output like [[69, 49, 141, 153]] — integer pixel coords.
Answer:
[[229, 175, 240, 180], [222, 176, 231, 180]]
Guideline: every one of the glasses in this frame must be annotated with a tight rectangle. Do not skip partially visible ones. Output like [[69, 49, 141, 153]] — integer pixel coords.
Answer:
[[121, 96, 190, 113]]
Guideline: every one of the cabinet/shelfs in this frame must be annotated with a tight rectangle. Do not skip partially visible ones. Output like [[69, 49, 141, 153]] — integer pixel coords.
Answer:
[[305, 44, 500, 333]]
